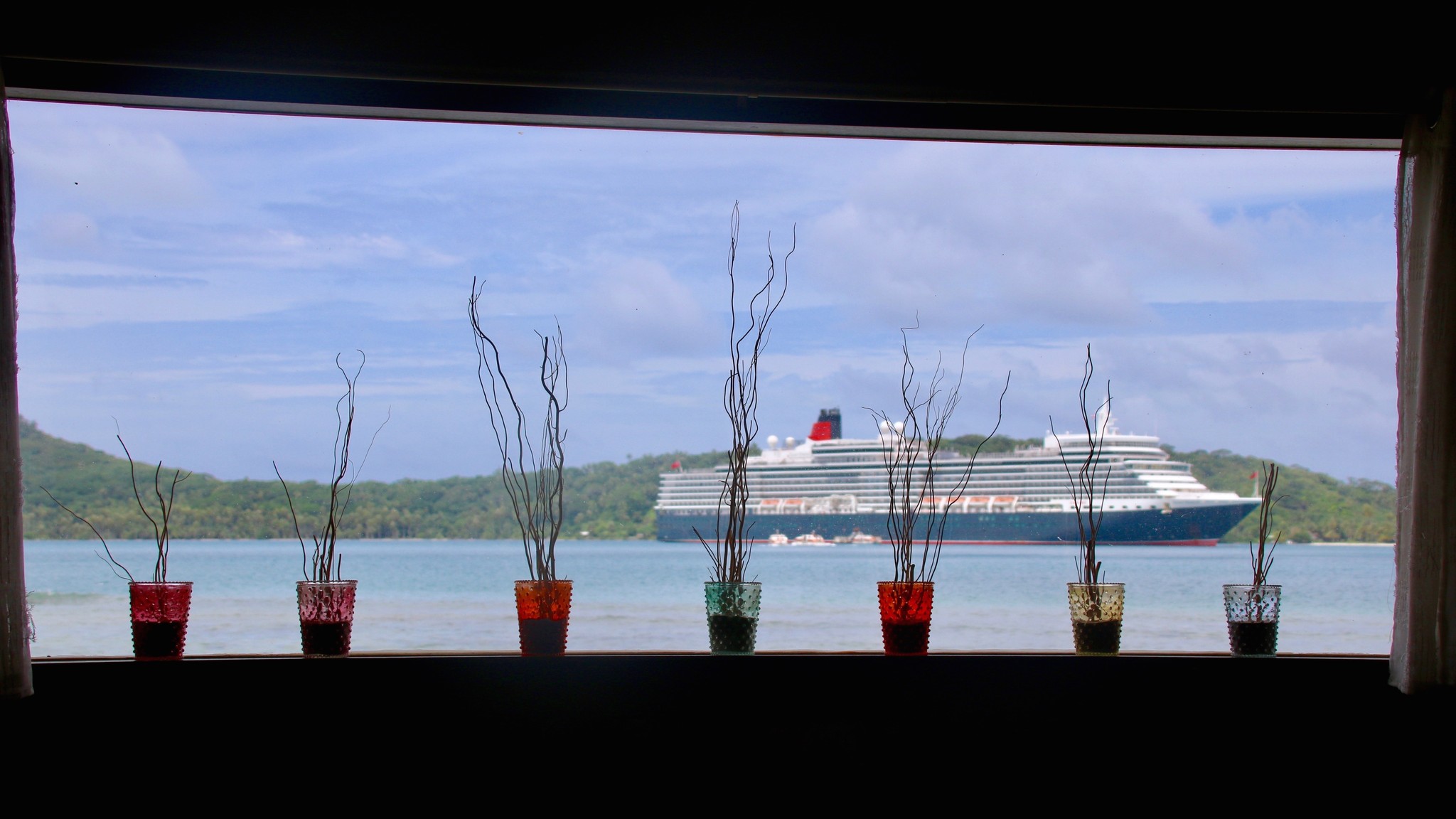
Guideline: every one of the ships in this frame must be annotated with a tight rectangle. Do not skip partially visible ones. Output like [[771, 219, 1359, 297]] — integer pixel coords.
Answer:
[[653, 406, 1263, 548]]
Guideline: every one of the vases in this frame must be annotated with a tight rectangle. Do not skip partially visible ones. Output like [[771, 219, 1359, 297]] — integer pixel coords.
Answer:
[[295, 580, 358, 658], [514, 579, 573, 655], [1067, 582, 1126, 656], [704, 582, 762, 655], [876, 581, 935, 655], [129, 580, 194, 660], [1223, 584, 1281, 652]]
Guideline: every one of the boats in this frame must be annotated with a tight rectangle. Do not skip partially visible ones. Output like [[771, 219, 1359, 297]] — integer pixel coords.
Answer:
[[943, 497, 966, 508], [989, 495, 1015, 510], [760, 499, 780, 510], [919, 496, 942, 510], [782, 499, 804, 510], [966, 496, 990, 510]]
